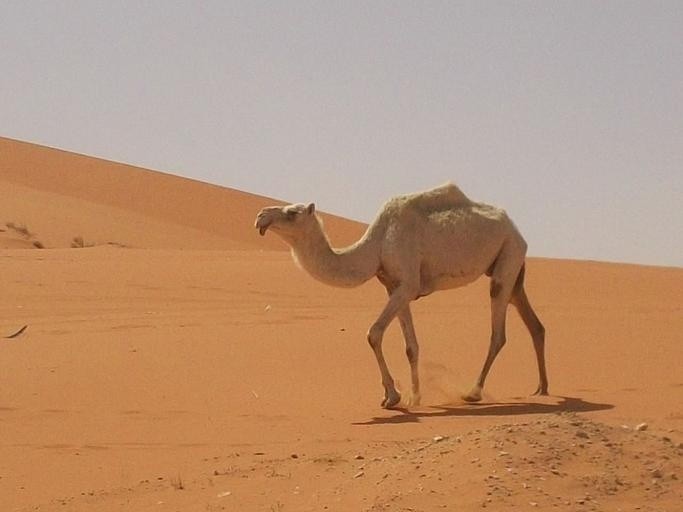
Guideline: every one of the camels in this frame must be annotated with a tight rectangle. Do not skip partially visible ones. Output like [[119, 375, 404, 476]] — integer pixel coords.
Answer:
[[254, 182, 549, 409]]
